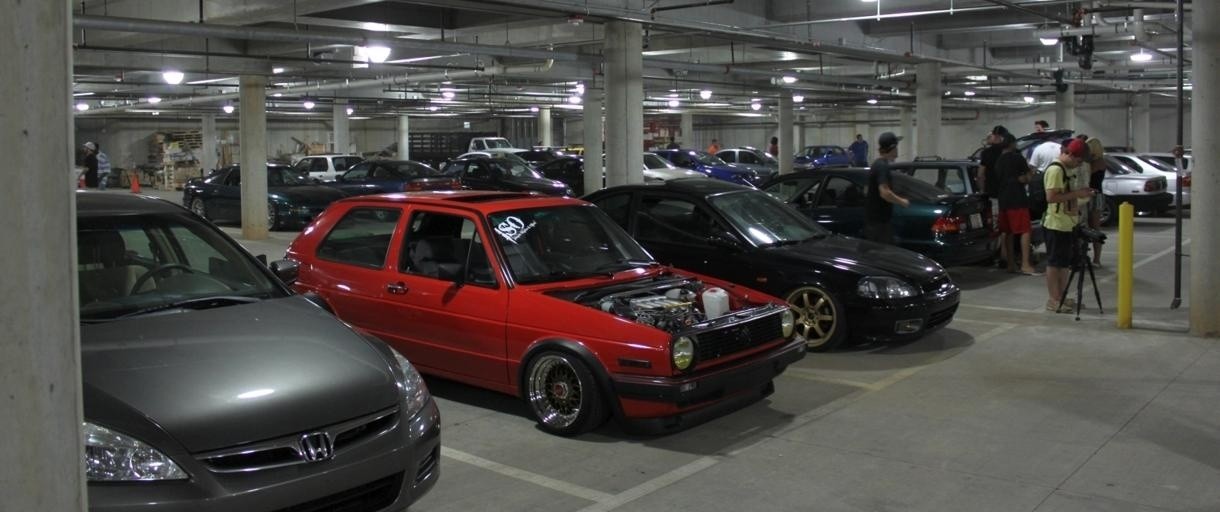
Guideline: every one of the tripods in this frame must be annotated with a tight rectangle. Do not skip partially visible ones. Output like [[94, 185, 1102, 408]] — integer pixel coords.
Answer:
[[1056, 242, 1103, 321]]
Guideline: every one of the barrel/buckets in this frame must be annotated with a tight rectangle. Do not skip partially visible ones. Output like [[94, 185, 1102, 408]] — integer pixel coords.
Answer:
[[702, 287, 730, 321]]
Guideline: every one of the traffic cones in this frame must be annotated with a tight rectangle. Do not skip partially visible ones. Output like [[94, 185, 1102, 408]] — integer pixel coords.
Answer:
[[128, 169, 143, 194]]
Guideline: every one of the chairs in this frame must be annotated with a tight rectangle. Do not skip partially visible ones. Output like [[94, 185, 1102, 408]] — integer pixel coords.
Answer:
[[83, 263, 157, 301], [501, 240, 552, 276], [414, 238, 455, 278]]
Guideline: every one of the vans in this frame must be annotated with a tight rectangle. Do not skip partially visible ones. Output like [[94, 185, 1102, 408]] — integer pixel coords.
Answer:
[[895, 128, 1079, 254]]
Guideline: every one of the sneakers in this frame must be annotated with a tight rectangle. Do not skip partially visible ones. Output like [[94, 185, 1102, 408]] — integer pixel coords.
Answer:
[[1047, 298, 1072, 314], [999, 260, 1045, 276]]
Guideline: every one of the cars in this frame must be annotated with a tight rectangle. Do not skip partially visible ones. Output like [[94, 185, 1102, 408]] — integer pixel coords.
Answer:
[[1142, 148, 1193, 172], [760, 163, 1005, 268], [1091, 153, 1177, 225], [567, 171, 967, 361], [1109, 149, 1191, 211], [273, 177, 818, 444], [76, 185, 445, 510]]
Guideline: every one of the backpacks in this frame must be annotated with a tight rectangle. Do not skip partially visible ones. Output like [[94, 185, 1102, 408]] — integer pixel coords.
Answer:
[[1030, 162, 1066, 220]]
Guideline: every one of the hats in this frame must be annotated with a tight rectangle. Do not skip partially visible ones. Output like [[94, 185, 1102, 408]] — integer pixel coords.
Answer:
[[83, 142, 97, 151], [1066, 138, 1090, 161], [879, 132, 903, 147]]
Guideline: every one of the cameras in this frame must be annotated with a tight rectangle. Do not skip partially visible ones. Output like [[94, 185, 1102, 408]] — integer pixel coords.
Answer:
[[1072, 221, 1107, 245]]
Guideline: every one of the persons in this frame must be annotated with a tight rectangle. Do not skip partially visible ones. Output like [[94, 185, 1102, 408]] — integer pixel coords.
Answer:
[[94, 142, 112, 190], [848, 134, 869, 167], [539, 141, 543, 146], [862, 133, 910, 245], [979, 121, 1108, 314], [768, 136, 777, 157], [666, 136, 678, 149], [708, 139, 719, 154], [75, 141, 97, 189]]
